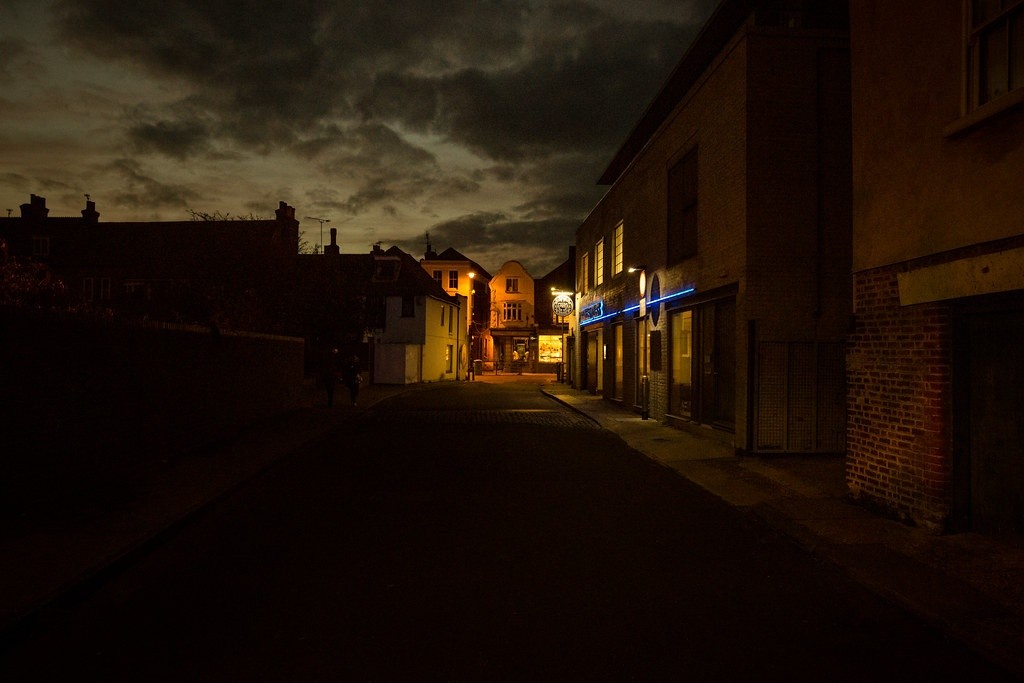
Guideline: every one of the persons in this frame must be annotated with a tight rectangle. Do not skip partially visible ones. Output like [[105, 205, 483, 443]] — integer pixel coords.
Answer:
[[347, 355, 362, 407], [500, 347, 530, 373]]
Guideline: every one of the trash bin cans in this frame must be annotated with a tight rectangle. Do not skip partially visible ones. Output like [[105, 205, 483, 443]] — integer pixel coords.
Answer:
[[474, 360, 482, 375]]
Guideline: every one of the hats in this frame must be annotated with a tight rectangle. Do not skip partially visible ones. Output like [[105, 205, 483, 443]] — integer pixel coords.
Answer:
[[351, 356, 360, 361]]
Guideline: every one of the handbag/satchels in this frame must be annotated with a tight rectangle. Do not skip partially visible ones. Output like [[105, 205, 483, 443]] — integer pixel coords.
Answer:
[[354, 376, 363, 384]]
